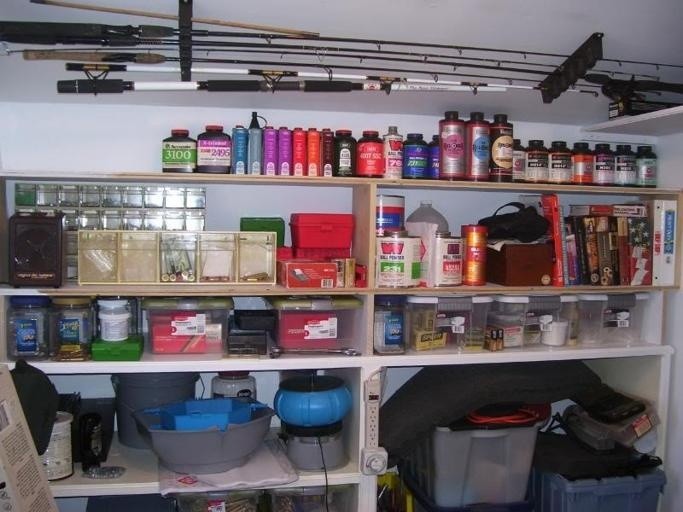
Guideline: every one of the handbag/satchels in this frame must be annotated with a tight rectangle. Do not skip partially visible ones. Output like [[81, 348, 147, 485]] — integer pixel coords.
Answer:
[[478, 202, 548, 243]]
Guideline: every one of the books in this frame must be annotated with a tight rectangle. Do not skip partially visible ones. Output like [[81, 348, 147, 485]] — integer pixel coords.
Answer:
[[157, 431, 299, 496]]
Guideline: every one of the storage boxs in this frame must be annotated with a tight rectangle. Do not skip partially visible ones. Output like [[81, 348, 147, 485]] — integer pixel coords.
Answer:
[[537, 466, 668, 512], [288, 211, 356, 249], [261, 296, 364, 357], [404, 293, 493, 351], [577, 291, 653, 350], [492, 293, 578, 353], [403, 402, 544, 511], [142, 296, 231, 357], [488, 236, 555, 287]]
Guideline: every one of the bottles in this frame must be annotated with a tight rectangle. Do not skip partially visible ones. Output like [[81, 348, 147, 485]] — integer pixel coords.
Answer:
[[49, 295, 94, 361], [98, 297, 131, 342], [374, 294, 405, 354], [5, 296, 54, 360], [210, 371, 257, 402]]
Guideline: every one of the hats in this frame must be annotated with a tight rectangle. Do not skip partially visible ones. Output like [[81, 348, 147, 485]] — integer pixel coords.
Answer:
[[10, 365, 58, 455]]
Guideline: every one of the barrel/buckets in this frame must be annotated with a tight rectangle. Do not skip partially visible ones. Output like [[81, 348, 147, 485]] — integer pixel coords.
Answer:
[[111, 374, 204, 450], [432, 237, 464, 284], [377, 237, 424, 287]]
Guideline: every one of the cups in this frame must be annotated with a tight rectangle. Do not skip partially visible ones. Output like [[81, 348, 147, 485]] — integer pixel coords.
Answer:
[[278, 127, 293, 176], [593, 143, 615, 186], [197, 125, 232, 174], [248, 111, 262, 176], [306, 128, 322, 176], [292, 127, 306, 176], [403, 132, 429, 180], [526, 140, 549, 183], [548, 141, 572, 184], [513, 138, 525, 182], [322, 127, 386, 178], [571, 142, 593, 185], [262, 126, 278, 175], [428, 111, 514, 183], [230, 124, 248, 175], [614, 144, 637, 187], [636, 145, 657, 188], [383, 125, 404, 179], [162, 128, 197, 174]]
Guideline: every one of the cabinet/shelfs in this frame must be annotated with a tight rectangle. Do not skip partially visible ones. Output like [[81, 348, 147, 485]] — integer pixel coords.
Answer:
[[371, 174, 681, 512], [2, 174, 375, 512]]
